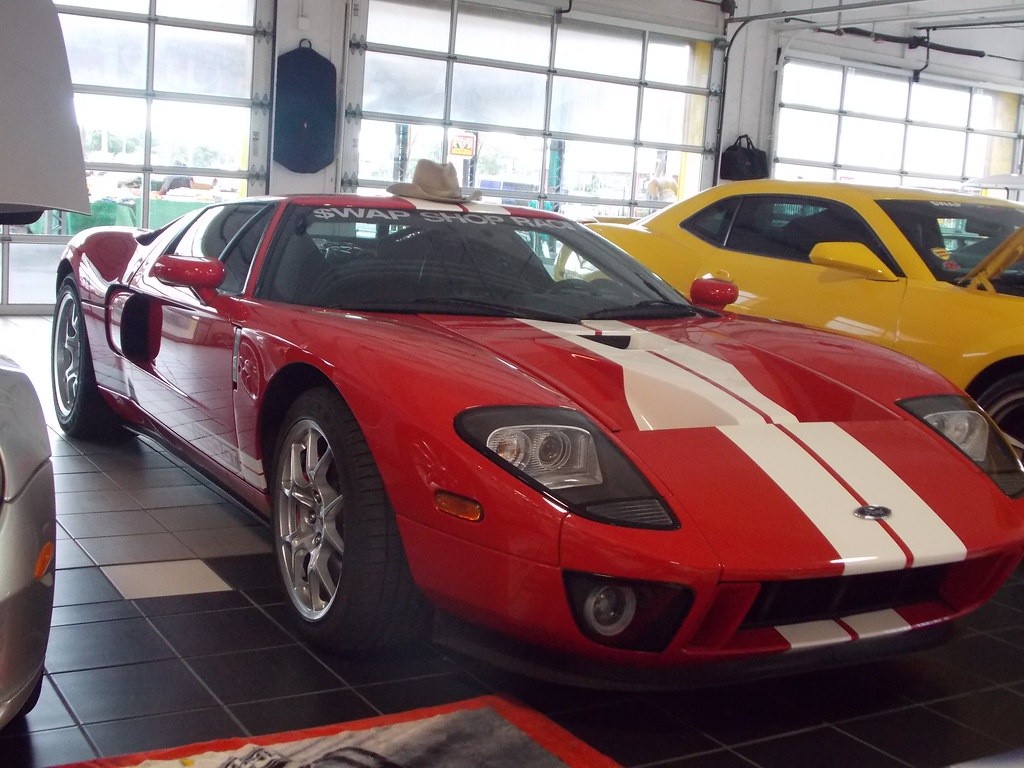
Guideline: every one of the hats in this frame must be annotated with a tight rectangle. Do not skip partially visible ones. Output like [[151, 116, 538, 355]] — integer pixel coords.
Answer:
[[386, 158, 470, 202]]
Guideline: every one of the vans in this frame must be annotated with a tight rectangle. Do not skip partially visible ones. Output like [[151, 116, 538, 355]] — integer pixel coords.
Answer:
[[474, 174, 586, 217]]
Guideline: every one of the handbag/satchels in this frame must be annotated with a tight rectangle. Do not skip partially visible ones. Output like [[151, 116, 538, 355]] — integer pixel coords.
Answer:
[[720, 136, 769, 181]]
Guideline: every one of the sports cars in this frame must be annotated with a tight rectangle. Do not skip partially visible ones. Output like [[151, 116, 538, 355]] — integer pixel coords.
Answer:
[[50, 193, 1023, 674], [553, 180, 1024, 447]]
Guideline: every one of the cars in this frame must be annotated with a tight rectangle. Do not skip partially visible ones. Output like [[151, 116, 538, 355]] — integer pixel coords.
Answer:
[[25, 159, 240, 237], [0, 0, 91, 730]]
